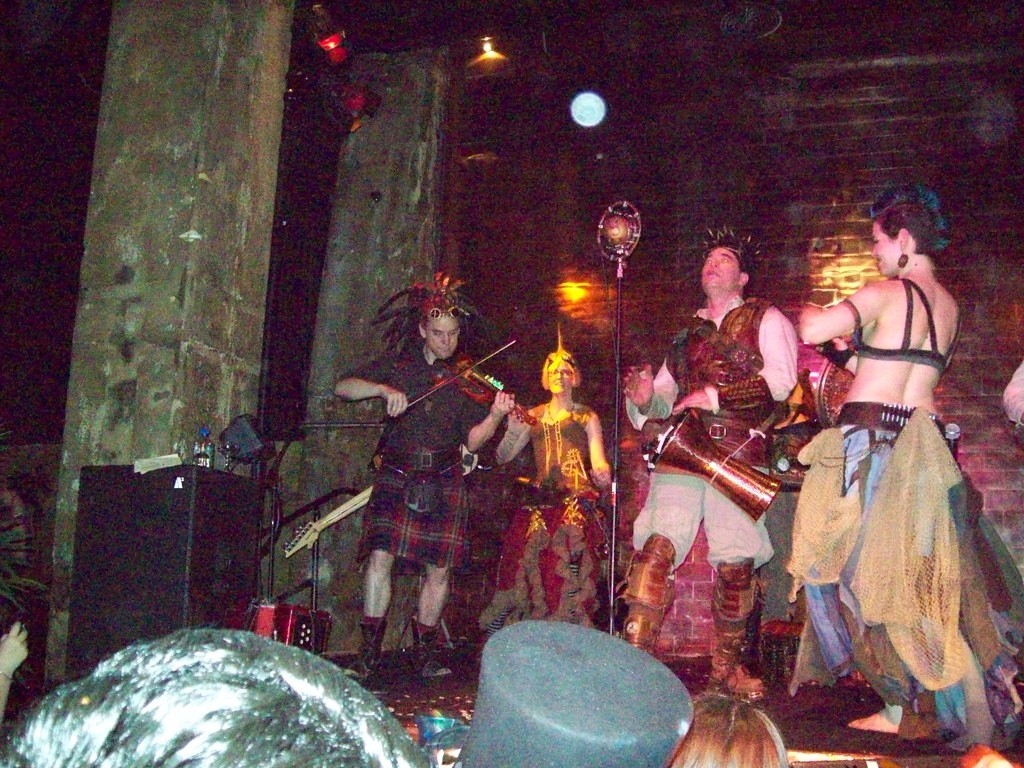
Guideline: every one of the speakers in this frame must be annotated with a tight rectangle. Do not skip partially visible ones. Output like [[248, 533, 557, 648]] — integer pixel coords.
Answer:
[[66, 464, 262, 687], [219, 413, 276, 463]]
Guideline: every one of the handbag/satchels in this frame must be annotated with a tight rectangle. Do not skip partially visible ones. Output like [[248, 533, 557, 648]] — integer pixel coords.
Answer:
[[404, 482, 445, 516]]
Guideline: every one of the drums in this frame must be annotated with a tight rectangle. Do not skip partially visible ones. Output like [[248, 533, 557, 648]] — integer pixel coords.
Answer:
[[647, 408, 783, 521]]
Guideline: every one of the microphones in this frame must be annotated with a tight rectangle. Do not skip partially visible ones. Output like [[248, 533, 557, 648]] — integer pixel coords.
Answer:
[[602, 212, 633, 246]]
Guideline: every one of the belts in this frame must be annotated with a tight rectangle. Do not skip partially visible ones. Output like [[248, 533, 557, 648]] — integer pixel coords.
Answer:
[[705, 424, 763, 459], [383, 448, 461, 469], [836, 402, 946, 439]]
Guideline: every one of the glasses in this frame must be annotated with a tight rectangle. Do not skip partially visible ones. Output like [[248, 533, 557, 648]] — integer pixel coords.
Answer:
[[430, 306, 461, 319], [547, 369, 573, 377], [702, 243, 742, 263]]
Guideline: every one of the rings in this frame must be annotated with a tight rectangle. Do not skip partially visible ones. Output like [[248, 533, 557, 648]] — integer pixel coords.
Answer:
[[510, 406, 514, 409], [631, 384, 636, 389], [500, 401, 504, 403]]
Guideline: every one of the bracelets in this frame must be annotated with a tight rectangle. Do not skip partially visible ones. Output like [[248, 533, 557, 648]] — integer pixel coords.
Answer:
[[0, 671, 13, 682]]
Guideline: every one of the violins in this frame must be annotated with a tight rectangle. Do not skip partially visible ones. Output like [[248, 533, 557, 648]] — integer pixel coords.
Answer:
[[431, 355, 538, 426]]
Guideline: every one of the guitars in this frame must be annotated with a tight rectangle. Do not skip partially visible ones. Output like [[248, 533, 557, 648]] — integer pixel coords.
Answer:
[[284, 484, 373, 558]]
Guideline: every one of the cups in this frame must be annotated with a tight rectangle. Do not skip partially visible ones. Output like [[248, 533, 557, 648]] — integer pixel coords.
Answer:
[[192, 425, 214, 468]]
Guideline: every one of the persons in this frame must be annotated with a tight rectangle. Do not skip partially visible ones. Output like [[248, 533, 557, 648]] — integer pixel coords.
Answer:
[[483, 352, 610, 638], [622, 238, 797, 696], [791, 187, 997, 748], [336, 277, 515, 694], [0, 622, 28, 723], [676, 691, 789, 768], [1, 629, 434, 768], [1004, 361, 1024, 425]]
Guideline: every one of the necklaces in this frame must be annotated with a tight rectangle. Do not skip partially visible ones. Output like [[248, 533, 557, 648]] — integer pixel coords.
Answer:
[[547, 403, 574, 422]]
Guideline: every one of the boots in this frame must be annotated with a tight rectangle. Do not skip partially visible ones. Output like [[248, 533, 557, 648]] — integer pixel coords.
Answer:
[[706, 559, 765, 701], [359, 616, 388, 693], [411, 613, 452, 677], [614, 551, 673, 657]]
